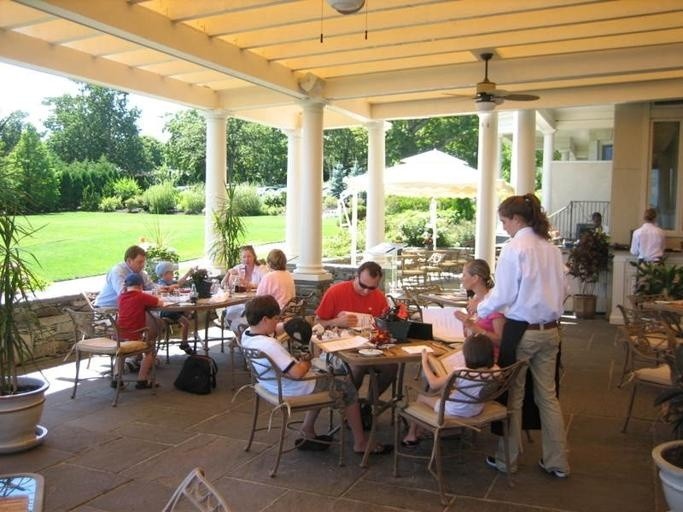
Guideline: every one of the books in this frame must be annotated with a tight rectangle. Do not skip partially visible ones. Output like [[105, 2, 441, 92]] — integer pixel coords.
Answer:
[[336, 311, 377, 333], [316, 337, 374, 353], [426, 349, 467, 380]]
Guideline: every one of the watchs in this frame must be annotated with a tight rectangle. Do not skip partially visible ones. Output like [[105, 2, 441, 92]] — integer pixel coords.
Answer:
[[402, 335, 496, 446]]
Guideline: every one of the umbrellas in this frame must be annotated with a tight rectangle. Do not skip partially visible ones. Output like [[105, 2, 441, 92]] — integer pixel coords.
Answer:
[[343, 148, 514, 249]]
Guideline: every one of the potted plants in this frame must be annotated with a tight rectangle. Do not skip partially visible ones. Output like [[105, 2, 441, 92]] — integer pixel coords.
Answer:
[[0, 112, 49, 454], [208, 176, 252, 327], [448, 199, 475, 247], [564, 229, 618, 319]]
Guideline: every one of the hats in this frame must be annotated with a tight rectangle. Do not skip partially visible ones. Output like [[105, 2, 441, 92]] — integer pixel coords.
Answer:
[[283, 318, 311, 345], [156, 262, 179, 278], [124, 272, 144, 284]]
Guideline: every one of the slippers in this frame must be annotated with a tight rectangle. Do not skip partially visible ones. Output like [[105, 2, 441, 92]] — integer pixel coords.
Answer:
[[111, 380, 125, 387], [399, 440, 418, 448], [136, 378, 159, 388], [297, 436, 331, 450], [357, 443, 392, 457]]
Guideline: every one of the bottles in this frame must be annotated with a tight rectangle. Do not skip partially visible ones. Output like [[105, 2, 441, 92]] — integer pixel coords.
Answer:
[[188, 283, 198, 304], [233, 276, 240, 292]]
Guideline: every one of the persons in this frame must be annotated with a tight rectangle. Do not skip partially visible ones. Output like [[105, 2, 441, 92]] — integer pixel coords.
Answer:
[[92, 245, 177, 387], [152, 260, 198, 354], [109, 273, 161, 390], [221, 246, 265, 346], [464, 194, 572, 482], [242, 295, 393, 456], [454, 258, 504, 363], [628, 209, 665, 263], [589, 211, 604, 238], [256, 249, 296, 308], [314, 261, 398, 428]]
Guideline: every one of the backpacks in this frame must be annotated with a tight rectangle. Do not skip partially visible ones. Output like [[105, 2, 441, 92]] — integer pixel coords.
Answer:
[[175, 355, 218, 395]]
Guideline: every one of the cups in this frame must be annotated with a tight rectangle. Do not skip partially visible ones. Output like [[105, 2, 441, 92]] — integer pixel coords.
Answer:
[[150, 288, 160, 298], [172, 290, 180, 305]]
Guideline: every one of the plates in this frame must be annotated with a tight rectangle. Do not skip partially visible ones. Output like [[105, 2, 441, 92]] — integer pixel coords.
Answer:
[[357, 347, 384, 357]]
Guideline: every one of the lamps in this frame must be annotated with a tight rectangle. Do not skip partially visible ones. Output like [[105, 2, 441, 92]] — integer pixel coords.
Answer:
[[320, 0, 368, 43], [473, 98, 505, 113]]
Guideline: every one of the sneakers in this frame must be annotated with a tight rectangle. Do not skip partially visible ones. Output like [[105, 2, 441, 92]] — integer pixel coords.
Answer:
[[180, 342, 194, 354], [486, 456, 497, 467], [538, 456, 571, 479]]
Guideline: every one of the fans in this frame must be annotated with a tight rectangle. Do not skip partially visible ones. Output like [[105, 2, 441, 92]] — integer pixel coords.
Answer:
[[443, 53, 542, 101]]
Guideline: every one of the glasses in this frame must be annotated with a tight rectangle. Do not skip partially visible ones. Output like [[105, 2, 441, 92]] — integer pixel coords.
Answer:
[[359, 272, 378, 290]]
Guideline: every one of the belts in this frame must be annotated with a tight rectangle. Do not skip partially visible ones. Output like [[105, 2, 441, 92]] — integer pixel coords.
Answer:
[[527, 321, 559, 330]]
